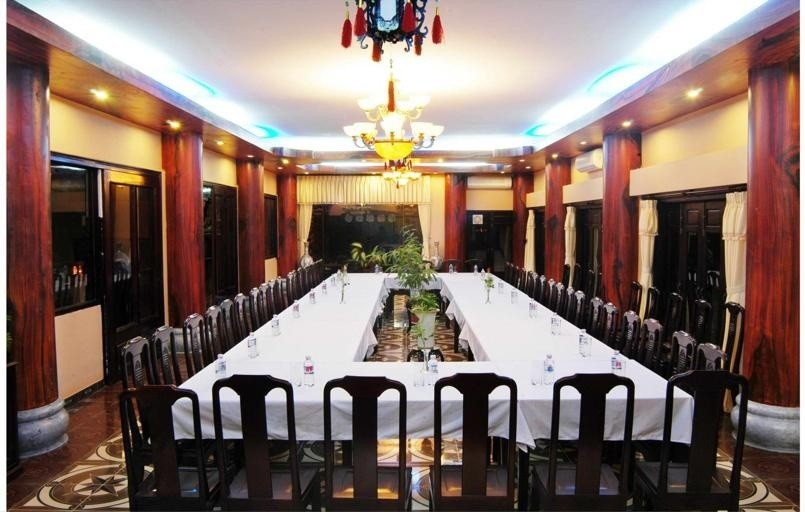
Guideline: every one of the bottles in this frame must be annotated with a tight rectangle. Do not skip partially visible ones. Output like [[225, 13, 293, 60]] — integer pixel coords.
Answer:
[[428, 354, 440, 385], [472, 263, 594, 359], [246, 269, 342, 358], [610, 350, 627, 377], [302, 356, 317, 388], [373, 263, 455, 276], [544, 354, 556, 387], [214, 354, 228, 378]]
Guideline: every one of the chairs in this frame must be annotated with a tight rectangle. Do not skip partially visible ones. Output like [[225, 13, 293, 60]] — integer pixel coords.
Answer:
[[118, 255, 329, 388], [506, 245, 752, 377], [340, 258, 488, 273]]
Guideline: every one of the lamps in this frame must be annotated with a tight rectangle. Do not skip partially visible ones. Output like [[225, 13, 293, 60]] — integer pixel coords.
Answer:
[[343, 1, 445, 59], [342, 70, 445, 185]]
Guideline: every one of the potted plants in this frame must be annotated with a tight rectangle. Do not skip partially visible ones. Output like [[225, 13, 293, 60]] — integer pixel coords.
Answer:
[[353, 221, 439, 354]]
